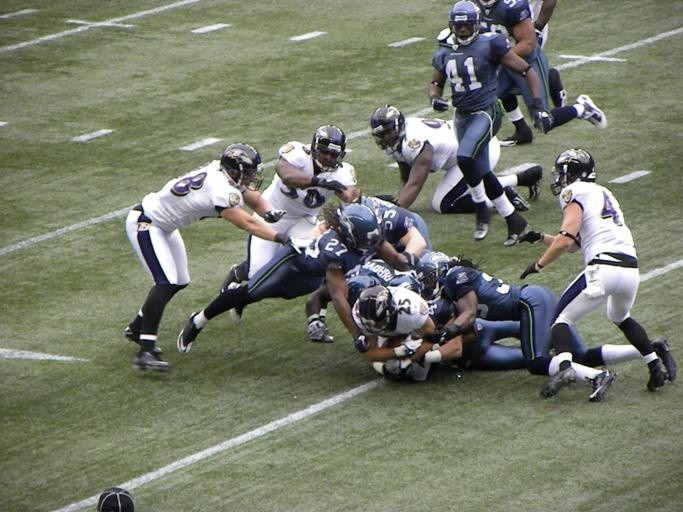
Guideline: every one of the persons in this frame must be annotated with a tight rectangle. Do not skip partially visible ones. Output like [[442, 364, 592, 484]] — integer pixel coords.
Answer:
[[516, 145, 666, 401], [221, 123, 363, 341], [177, 200, 416, 353], [470, 0, 607, 146], [425, 0, 547, 244], [122, 141, 292, 373], [370, 104, 547, 212], [528, 0, 567, 109], [306, 194, 526, 384], [418, 252, 677, 404]]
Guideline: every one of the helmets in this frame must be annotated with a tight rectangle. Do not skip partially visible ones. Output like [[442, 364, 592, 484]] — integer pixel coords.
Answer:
[[220, 143, 264, 191], [410, 251, 451, 301], [551, 148, 596, 195], [358, 285, 395, 337], [311, 125, 346, 172], [371, 105, 406, 155], [449, 1, 480, 46], [339, 203, 382, 256], [388, 275, 420, 295], [347, 275, 375, 305]]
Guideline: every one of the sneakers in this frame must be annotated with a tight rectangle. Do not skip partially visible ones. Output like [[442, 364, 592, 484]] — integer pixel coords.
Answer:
[[499, 127, 532, 147], [575, 95, 607, 129], [647, 362, 669, 391], [228, 282, 243, 325], [652, 339, 677, 381], [311, 335, 333, 342], [177, 312, 203, 355], [585, 367, 618, 402], [504, 224, 531, 247], [549, 68, 567, 108], [131, 351, 170, 372], [525, 162, 544, 201], [474, 223, 489, 240], [540, 366, 576, 398], [124, 323, 163, 355], [503, 185, 531, 211], [220, 264, 242, 294]]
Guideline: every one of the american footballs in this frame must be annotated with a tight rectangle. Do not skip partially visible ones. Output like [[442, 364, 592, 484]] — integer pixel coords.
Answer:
[[382, 334, 419, 348]]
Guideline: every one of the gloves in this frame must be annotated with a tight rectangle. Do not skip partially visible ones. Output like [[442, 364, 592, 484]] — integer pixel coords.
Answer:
[[307, 313, 328, 342], [354, 333, 370, 352], [534, 98, 551, 134], [430, 95, 448, 112], [265, 209, 287, 223], [393, 335, 423, 357], [518, 231, 544, 244], [274, 232, 315, 255], [311, 177, 347, 193], [521, 261, 543, 279], [422, 324, 462, 344]]
